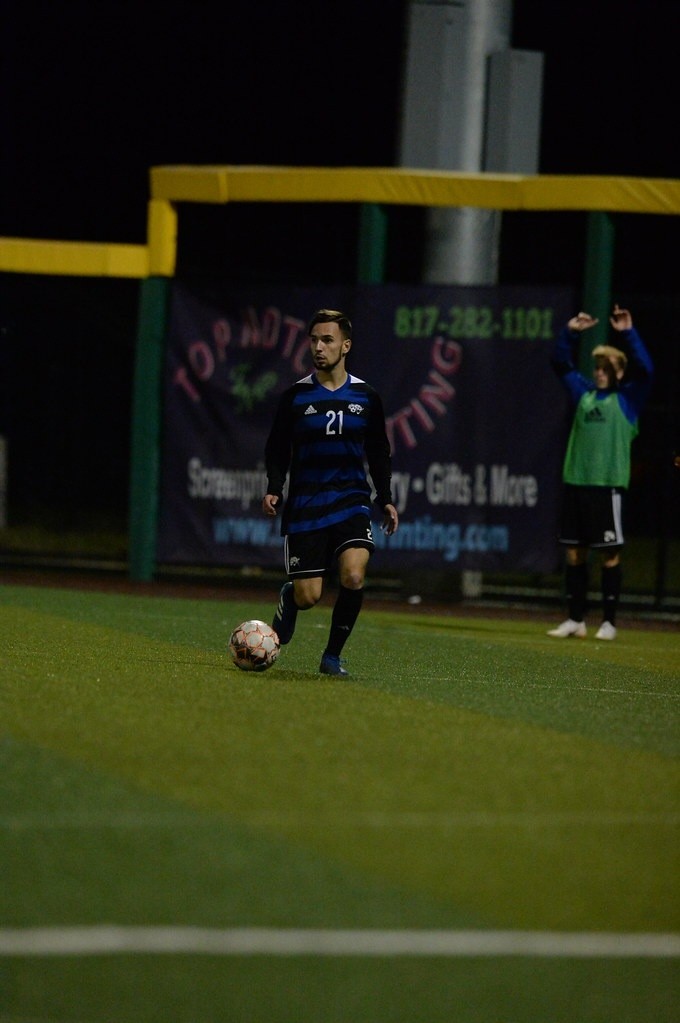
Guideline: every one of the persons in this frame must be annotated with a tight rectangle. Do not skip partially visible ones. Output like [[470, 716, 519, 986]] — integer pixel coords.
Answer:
[[263, 310, 398, 675], [544, 302, 653, 641]]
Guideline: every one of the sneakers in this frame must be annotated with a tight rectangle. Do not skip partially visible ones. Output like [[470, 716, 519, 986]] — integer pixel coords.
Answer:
[[272, 581, 298, 646], [546, 619, 586, 639], [319, 654, 348, 675], [594, 621, 616, 641]]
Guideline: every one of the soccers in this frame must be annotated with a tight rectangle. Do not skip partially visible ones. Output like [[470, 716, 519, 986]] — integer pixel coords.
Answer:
[[228, 619, 280, 670]]
